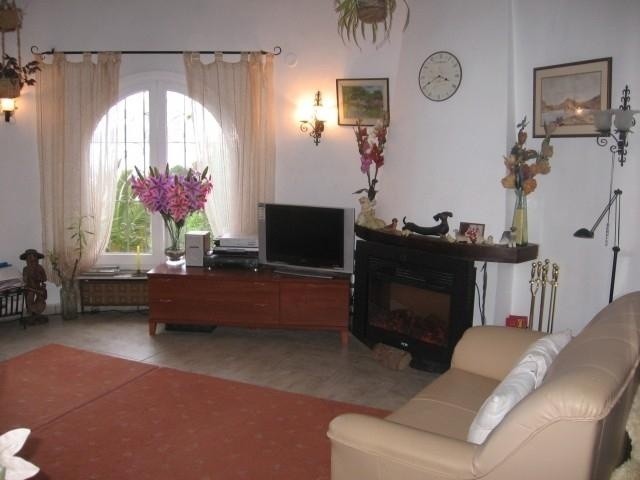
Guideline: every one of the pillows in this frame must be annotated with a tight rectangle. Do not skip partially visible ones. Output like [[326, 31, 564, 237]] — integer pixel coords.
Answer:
[[468, 330, 572, 445]]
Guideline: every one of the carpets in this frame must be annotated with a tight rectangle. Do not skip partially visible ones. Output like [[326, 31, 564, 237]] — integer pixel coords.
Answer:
[[1, 342, 392, 479]]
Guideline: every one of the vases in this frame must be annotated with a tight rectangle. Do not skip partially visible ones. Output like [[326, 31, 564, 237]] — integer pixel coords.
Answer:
[[509, 193, 529, 247], [356, 197, 387, 230], [59, 279, 79, 319], [164, 239, 186, 267]]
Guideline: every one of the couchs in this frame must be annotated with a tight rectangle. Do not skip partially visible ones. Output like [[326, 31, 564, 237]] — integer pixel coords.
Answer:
[[329, 293, 639, 478]]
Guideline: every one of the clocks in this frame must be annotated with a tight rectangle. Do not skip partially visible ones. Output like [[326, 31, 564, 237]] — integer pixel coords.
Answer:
[[418, 51, 463, 102]]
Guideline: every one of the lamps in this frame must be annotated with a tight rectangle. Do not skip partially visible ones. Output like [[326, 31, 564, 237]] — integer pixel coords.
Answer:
[[295, 91, 337, 146], [573, 188, 623, 303], [0, 98, 16, 122]]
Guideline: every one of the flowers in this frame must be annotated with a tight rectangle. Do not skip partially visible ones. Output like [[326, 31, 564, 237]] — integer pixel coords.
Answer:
[[46, 216, 94, 286], [129, 162, 213, 243], [501, 115, 557, 192], [351, 114, 387, 201]]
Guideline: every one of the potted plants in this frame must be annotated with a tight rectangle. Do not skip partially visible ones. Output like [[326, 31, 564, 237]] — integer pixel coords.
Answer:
[[1, 54, 42, 95], [333, 0, 410, 50]]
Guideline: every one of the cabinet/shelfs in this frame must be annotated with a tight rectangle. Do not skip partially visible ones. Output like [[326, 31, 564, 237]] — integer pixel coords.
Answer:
[[78, 266, 147, 317], [147, 266, 353, 343]]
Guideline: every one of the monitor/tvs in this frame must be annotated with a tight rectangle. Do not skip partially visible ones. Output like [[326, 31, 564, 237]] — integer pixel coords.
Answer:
[[256, 200, 356, 279]]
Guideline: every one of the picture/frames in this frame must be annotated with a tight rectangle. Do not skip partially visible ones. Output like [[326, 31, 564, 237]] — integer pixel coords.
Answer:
[[336, 78, 390, 126], [534, 57, 612, 137]]
